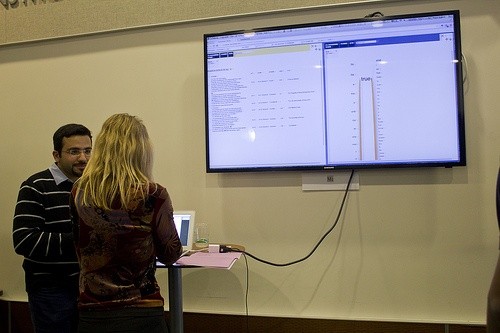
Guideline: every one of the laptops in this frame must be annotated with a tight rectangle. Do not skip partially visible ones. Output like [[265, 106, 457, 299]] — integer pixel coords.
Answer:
[[155, 210, 195, 261]]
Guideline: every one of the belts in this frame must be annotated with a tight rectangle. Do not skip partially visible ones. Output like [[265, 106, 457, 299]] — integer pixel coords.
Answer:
[[79, 310, 162, 317]]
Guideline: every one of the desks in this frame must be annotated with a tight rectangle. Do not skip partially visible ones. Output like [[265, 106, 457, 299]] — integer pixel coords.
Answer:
[[124, 243, 244, 333]]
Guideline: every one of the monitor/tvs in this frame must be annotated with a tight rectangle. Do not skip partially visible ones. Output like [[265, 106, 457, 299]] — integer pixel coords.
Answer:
[[204, 9, 467, 173]]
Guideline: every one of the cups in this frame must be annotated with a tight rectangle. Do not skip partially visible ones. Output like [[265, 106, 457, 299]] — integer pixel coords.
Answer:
[[196, 223, 209, 248]]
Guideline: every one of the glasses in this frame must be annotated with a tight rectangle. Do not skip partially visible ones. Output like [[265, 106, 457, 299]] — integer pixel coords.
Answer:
[[60, 149, 92, 157]]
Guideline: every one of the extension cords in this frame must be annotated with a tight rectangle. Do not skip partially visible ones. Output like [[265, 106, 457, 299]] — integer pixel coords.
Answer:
[[208, 243, 220, 253]]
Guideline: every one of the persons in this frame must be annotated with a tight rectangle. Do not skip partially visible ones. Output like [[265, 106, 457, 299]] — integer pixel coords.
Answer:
[[70, 114, 182, 333], [13, 124, 93, 333]]
